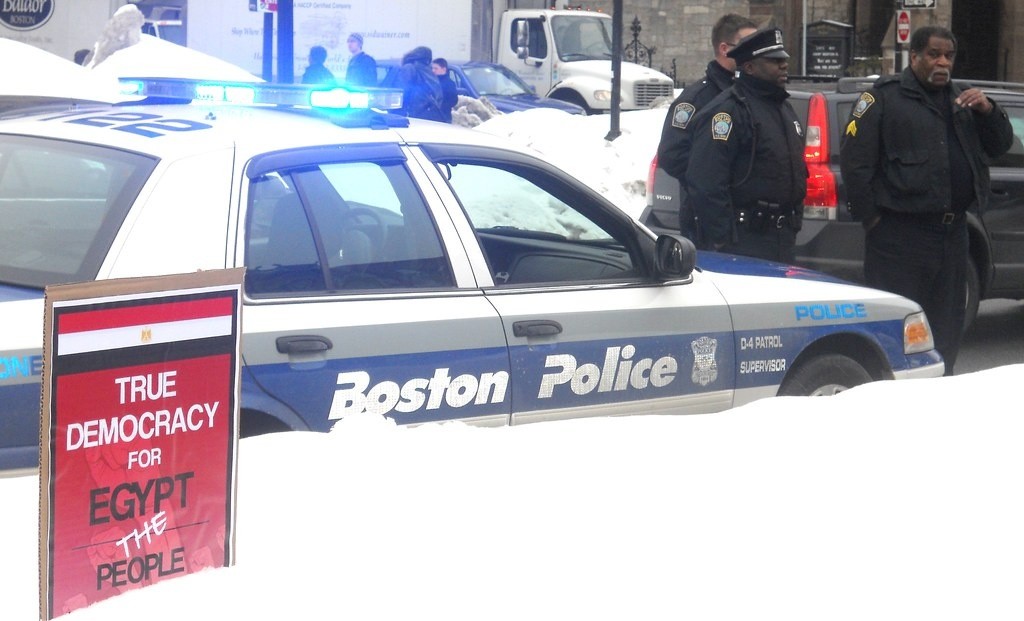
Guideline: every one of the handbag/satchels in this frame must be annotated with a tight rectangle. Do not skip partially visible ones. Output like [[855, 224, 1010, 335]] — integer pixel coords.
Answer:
[[410, 70, 447, 123]]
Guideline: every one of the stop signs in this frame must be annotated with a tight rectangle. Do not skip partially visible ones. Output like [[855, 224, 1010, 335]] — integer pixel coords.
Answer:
[[896, 9, 911, 45]]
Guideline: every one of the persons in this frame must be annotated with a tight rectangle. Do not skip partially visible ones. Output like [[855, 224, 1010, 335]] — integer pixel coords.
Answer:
[[346, 32, 377, 88], [431, 58, 458, 124], [378, 46, 444, 122], [301, 45, 337, 85], [653, 13, 759, 247], [679, 25, 809, 266], [840, 26, 1014, 376]]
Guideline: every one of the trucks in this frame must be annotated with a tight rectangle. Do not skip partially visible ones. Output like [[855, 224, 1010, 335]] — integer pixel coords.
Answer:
[[185, 1, 674, 114]]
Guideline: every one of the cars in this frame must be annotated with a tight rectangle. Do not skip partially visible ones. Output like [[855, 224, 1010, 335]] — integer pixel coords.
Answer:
[[1, 75, 945, 473], [642, 76, 1024, 328], [373, 60, 587, 117]]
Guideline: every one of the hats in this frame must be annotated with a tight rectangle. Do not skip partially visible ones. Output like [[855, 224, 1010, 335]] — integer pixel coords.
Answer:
[[726, 26, 791, 66], [349, 32, 363, 46]]
